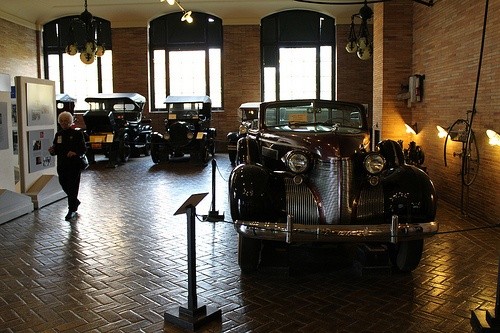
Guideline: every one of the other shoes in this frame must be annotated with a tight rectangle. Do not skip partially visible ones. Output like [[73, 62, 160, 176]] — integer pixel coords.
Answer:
[[66, 211, 73, 220], [75, 198, 80, 211]]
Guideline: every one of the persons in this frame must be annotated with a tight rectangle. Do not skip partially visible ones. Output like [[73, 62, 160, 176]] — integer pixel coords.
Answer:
[[48, 111, 87, 222]]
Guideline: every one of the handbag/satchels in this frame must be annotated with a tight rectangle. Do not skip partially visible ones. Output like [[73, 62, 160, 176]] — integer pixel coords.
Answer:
[[80, 153, 89, 170]]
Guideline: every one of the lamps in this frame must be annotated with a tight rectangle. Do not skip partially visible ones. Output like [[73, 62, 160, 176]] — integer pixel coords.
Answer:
[[167, 0, 194, 23], [65, 1, 105, 64], [436, 125, 452, 140], [486, 130, 500, 147], [239, 125, 247, 133], [346, 0, 374, 60], [405, 122, 418, 136]]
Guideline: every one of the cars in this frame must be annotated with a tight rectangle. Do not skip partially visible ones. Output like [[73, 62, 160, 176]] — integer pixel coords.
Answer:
[[150, 96, 218, 170], [227, 101, 263, 163], [227, 98, 439, 275], [83, 97, 166, 164]]
